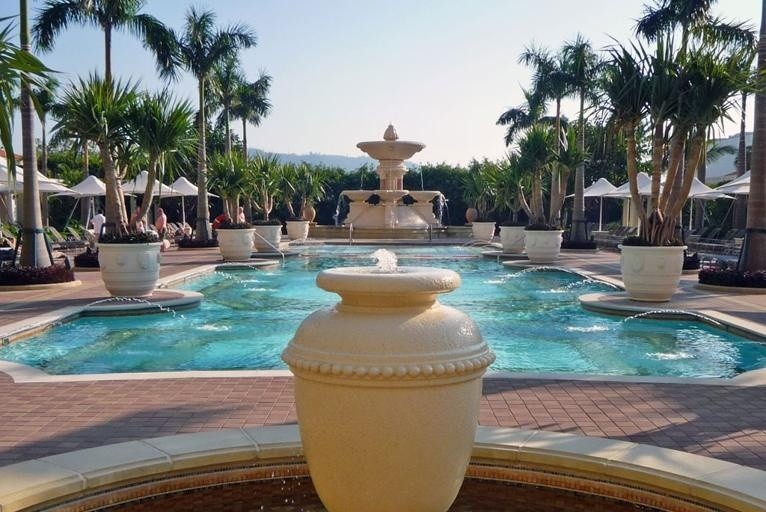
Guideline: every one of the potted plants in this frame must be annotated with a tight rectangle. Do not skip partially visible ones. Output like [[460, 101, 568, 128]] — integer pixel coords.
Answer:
[[278, 157, 332, 240], [455, 155, 501, 244], [245, 151, 298, 253], [481, 149, 531, 256], [515, 113, 591, 265], [201, 149, 268, 262], [48, 66, 202, 300], [583, 0, 766, 303]]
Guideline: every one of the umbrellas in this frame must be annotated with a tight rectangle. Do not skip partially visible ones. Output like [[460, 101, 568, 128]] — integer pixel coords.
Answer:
[[121, 170, 183, 197], [604, 172, 738, 235], [565, 176, 630, 232], [50, 175, 138, 214], [0, 156, 80, 197], [696, 169, 751, 197], [159, 176, 220, 223]]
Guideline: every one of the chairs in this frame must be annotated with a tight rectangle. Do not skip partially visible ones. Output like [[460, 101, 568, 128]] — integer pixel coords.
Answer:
[[0, 218, 197, 262], [587, 218, 746, 273]]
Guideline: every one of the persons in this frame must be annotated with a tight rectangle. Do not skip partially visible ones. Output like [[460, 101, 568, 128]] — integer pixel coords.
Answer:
[[239, 207, 246, 223], [130, 206, 147, 235], [90, 206, 106, 246], [155, 208, 167, 252], [224, 215, 231, 224], [174, 227, 191, 239], [212, 211, 225, 229], [0, 223, 16, 249]]
